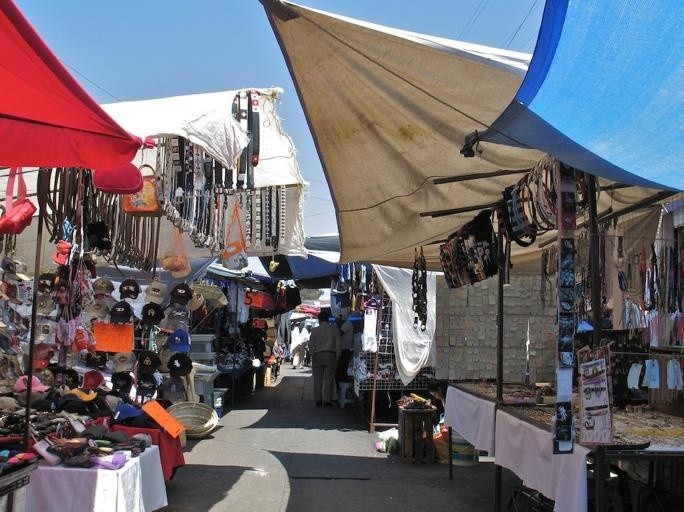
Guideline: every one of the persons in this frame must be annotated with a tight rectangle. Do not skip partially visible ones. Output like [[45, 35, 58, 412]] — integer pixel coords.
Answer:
[[286, 310, 353, 407]]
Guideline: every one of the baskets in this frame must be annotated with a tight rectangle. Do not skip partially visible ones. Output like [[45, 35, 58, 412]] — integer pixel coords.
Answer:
[[185, 279, 229, 312], [163, 400, 220, 439]]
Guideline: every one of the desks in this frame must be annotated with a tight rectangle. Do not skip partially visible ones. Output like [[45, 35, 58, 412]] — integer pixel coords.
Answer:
[[109, 423, 186, 482], [24, 445, 169, 512], [443, 382, 684, 512]]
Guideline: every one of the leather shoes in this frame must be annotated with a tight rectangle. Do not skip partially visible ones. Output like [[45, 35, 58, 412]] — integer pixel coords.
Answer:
[[316, 400, 334, 408]]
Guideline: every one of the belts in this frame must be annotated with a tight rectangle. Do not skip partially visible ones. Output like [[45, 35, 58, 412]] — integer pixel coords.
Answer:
[[498, 149, 601, 249], [36, 165, 155, 274], [154, 91, 285, 273]]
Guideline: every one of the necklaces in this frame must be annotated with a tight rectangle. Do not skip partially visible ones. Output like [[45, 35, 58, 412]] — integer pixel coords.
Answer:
[[627, 242, 683, 314]]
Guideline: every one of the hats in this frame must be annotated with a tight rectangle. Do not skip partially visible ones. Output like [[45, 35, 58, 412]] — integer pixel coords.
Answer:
[[134, 374, 158, 398], [117, 278, 140, 300], [162, 379, 188, 405], [143, 281, 167, 306], [111, 372, 133, 394], [112, 401, 144, 422], [109, 300, 132, 324], [112, 350, 136, 374], [94, 440, 114, 453], [160, 308, 190, 330], [166, 352, 193, 378], [103, 430, 137, 450], [0, 240, 114, 419], [169, 282, 193, 306], [136, 349, 161, 379], [140, 301, 166, 326], [164, 328, 191, 353]]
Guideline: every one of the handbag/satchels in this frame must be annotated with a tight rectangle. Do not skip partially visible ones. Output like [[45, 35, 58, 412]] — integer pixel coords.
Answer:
[[161, 253, 192, 279], [65, 452, 98, 468], [37, 429, 89, 459], [27, 424, 63, 466], [0, 197, 36, 236]]
